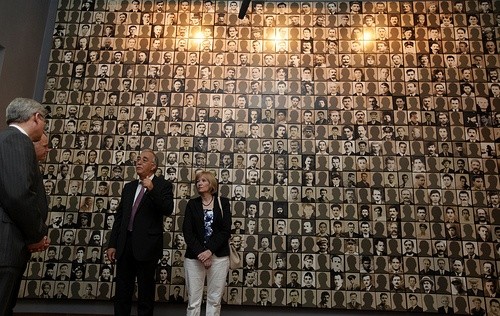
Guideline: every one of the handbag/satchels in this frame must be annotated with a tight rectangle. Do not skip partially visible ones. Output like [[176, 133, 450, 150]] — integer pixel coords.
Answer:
[[228, 238, 241, 268]]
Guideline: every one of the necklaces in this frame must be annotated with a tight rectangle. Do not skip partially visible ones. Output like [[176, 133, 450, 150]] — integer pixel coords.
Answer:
[[202, 195, 213, 206]]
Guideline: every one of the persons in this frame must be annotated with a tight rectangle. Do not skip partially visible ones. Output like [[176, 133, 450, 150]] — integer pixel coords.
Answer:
[[0, 98, 51, 316], [107, 149, 174, 316], [13, 0, 500, 316], [33, 131, 50, 161], [182, 171, 232, 316]]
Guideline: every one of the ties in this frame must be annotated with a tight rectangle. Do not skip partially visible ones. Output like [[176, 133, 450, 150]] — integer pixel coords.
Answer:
[[128, 182, 145, 231]]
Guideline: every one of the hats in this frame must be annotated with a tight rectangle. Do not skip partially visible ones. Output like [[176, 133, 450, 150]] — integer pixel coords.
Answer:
[[261, 187, 270, 192], [225, 80, 235, 84], [316, 238, 329, 245], [195, 152, 206, 159], [80, 213, 90, 219], [420, 276, 433, 286], [346, 239, 356, 244], [441, 159, 451, 164], [55, 24, 66, 30], [382, 127, 393, 132], [113, 166, 122, 172]]
